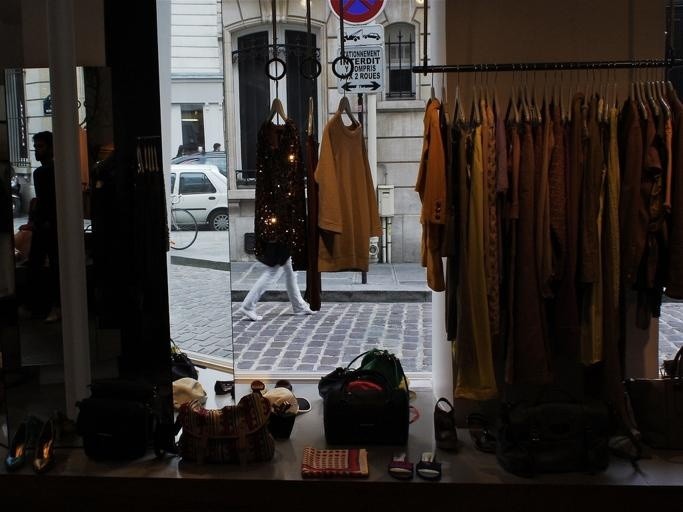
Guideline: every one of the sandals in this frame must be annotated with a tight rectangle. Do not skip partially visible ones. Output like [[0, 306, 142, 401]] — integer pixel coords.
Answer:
[[468, 414, 496, 452], [434, 398, 457, 449]]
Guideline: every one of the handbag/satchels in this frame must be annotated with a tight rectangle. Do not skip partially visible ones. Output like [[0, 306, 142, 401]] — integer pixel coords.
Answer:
[[623, 378, 683, 449], [179, 392, 275, 465], [171, 353, 197, 381], [319, 348, 408, 446]]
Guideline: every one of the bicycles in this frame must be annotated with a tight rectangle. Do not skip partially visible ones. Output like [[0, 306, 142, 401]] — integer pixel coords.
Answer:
[[171, 194, 197, 250]]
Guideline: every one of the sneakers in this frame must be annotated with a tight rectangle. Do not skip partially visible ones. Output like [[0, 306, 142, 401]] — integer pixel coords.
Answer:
[[240, 307, 262, 321], [295, 306, 317, 316]]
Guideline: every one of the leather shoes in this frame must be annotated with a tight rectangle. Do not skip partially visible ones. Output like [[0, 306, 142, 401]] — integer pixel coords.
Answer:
[[6, 422, 27, 469], [33, 419, 55, 473]]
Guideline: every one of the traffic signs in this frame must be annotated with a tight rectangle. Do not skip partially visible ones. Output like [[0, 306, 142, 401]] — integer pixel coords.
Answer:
[[338, 25, 384, 96]]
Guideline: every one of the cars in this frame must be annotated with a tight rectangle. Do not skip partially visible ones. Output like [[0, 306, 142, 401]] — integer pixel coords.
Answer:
[[170, 152, 228, 230]]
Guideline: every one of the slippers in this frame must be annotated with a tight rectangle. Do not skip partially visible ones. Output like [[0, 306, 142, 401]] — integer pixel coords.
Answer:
[[416, 452, 441, 478], [389, 451, 413, 479]]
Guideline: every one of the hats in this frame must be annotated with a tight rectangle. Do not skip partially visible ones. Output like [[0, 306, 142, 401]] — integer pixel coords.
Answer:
[[264, 380, 312, 414], [173, 377, 206, 409]]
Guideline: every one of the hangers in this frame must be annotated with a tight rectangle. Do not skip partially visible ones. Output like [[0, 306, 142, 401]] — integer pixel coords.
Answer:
[[266, 76, 287, 123], [430, 55, 683, 126], [339, 73, 359, 128]]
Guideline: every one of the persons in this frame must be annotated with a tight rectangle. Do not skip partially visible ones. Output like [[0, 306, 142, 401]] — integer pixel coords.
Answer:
[[238, 257, 318, 322], [212, 144, 221, 151], [21, 131, 61, 321], [176, 144, 184, 156]]
